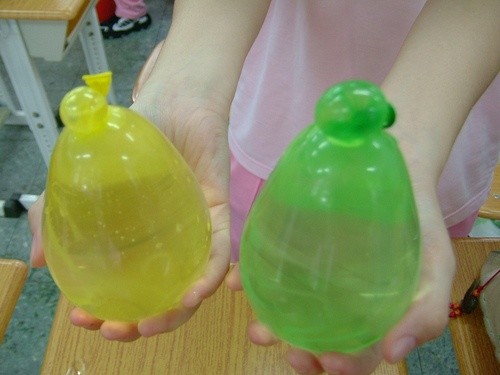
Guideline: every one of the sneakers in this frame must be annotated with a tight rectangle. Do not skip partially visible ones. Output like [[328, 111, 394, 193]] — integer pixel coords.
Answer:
[[99, 13, 152, 39]]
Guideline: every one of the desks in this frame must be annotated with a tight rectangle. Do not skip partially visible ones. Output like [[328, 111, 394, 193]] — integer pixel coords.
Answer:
[[0, 0, 500, 375]]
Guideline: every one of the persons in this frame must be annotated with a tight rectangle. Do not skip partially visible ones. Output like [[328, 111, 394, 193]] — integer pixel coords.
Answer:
[[28, 0, 500, 375], [100, 0, 152, 40]]
